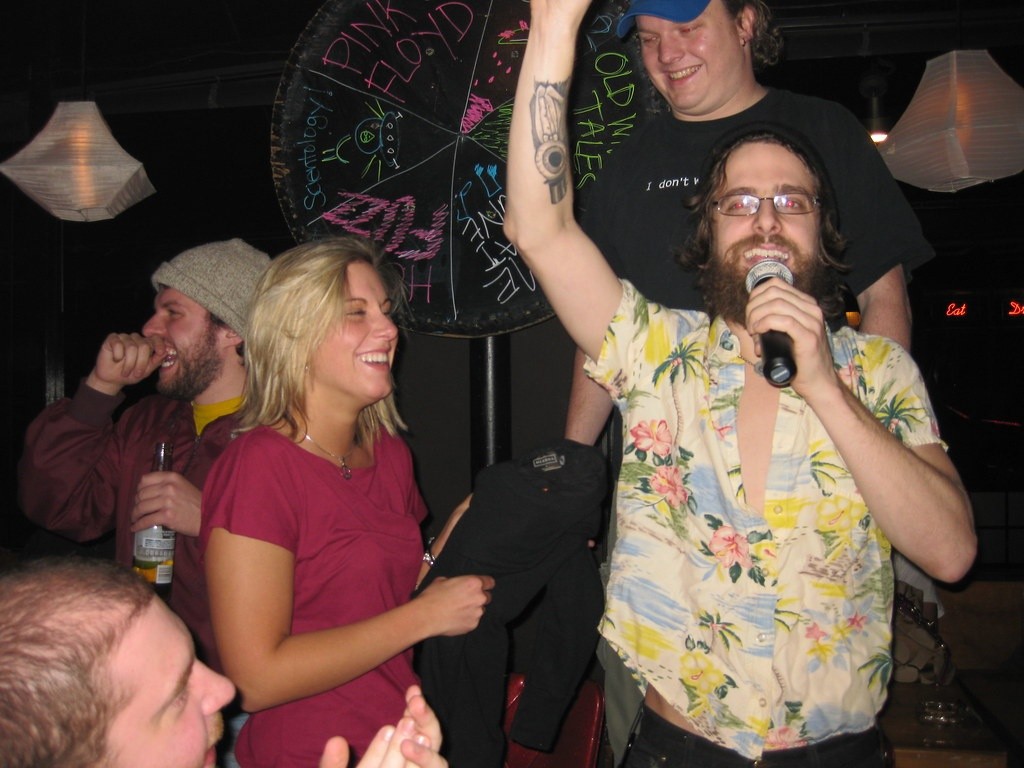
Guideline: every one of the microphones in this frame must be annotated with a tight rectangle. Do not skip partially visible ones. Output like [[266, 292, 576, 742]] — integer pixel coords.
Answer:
[[745, 259, 798, 388]]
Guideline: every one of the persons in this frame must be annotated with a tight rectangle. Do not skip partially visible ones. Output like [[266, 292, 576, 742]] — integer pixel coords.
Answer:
[[0, 237, 497, 768], [502, 0, 979, 768]]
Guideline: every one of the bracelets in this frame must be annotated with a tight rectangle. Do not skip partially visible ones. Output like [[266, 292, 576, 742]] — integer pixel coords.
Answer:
[[423, 536, 436, 567]]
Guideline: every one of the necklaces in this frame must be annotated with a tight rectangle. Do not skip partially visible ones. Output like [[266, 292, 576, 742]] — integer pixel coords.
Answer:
[[296, 424, 357, 481], [737, 353, 764, 377]]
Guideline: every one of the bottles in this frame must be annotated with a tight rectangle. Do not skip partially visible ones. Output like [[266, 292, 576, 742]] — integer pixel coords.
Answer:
[[132, 441, 176, 594]]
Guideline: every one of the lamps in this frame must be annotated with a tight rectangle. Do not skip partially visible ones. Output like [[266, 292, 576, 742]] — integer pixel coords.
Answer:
[[0, 102, 154, 219], [877, 50, 1024, 193]]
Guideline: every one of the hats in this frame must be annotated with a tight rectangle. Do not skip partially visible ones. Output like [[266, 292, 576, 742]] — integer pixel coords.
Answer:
[[150, 239, 272, 342], [616, 0, 711, 39]]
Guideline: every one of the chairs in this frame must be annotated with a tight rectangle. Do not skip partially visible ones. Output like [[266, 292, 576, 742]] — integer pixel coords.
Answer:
[[500, 672, 605, 768]]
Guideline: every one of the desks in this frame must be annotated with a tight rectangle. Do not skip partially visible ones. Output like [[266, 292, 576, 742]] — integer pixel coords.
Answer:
[[876, 677, 1008, 768]]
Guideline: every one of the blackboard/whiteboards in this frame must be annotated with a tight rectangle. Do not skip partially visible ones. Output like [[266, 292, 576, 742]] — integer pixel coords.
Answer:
[[267, 0, 689, 344]]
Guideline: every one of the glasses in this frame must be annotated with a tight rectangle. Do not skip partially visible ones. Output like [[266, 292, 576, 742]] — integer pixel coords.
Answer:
[[712, 193, 822, 216]]
